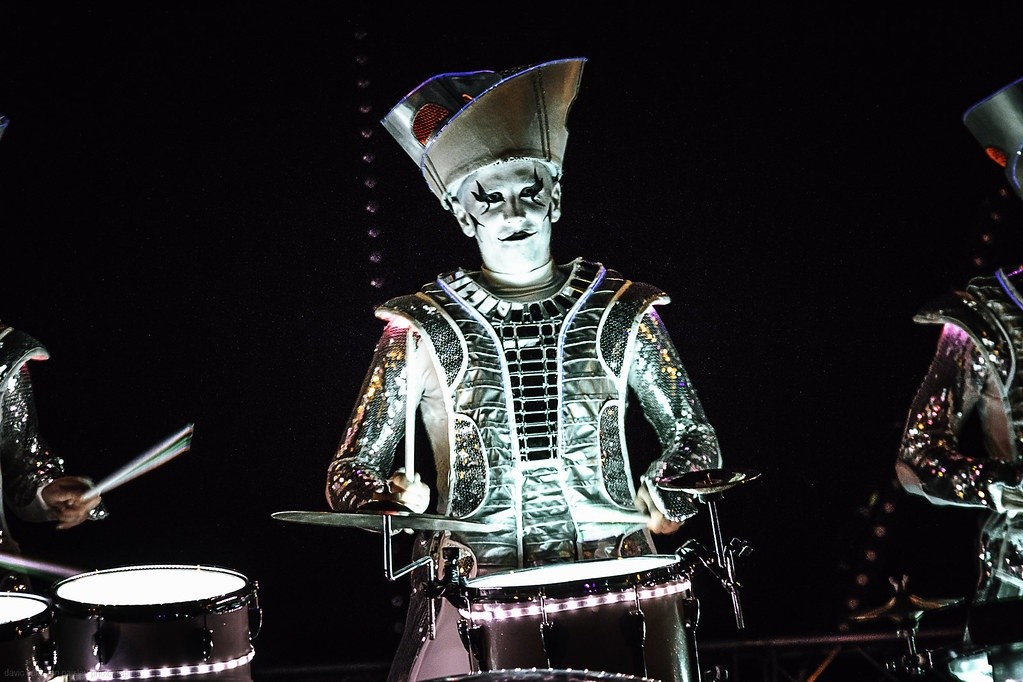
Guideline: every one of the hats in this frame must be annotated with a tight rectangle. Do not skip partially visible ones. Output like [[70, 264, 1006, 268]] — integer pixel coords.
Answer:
[[962, 79, 1023, 197], [379, 57, 587, 214]]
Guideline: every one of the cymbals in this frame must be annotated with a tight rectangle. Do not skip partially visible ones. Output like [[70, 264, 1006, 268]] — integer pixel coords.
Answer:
[[270, 510, 501, 532], [656, 468, 764, 494], [851, 592, 967, 628]]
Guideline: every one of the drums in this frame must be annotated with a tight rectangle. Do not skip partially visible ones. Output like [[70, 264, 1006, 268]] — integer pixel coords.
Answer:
[[403, 667, 664, 682], [52, 564, 258, 682], [938, 596, 1023, 682], [0, 591, 52, 682], [456, 551, 703, 681]]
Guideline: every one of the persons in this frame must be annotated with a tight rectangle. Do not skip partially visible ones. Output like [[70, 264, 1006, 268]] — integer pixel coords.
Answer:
[[895, 73, 1023, 677], [0, 320, 110, 682], [322, 60, 725, 682]]
